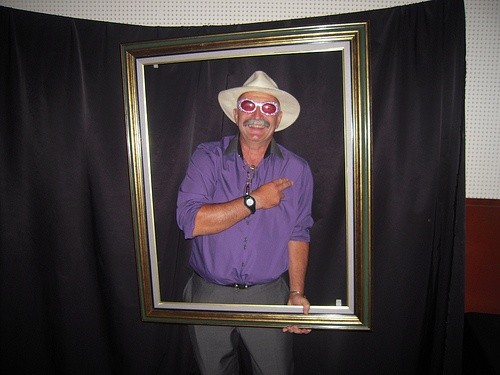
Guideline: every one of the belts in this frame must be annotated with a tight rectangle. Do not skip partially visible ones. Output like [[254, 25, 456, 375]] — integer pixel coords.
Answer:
[[193, 270, 256, 289]]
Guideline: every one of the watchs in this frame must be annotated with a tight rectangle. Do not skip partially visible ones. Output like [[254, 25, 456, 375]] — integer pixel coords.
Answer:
[[244, 194, 258, 215]]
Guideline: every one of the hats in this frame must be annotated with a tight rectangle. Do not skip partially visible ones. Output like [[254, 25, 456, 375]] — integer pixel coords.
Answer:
[[217, 71, 300, 132]]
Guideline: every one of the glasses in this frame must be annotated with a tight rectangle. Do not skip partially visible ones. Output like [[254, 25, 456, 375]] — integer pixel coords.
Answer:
[[237, 98, 281, 116]]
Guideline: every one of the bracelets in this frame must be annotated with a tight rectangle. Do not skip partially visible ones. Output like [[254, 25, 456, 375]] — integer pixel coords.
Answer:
[[290, 291, 303, 294]]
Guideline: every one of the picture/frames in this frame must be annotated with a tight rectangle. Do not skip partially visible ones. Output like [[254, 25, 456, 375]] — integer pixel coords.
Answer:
[[122, 21, 371, 330]]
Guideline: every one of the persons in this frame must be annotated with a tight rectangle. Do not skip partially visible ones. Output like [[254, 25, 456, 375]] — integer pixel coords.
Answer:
[[175, 69, 314, 375]]
